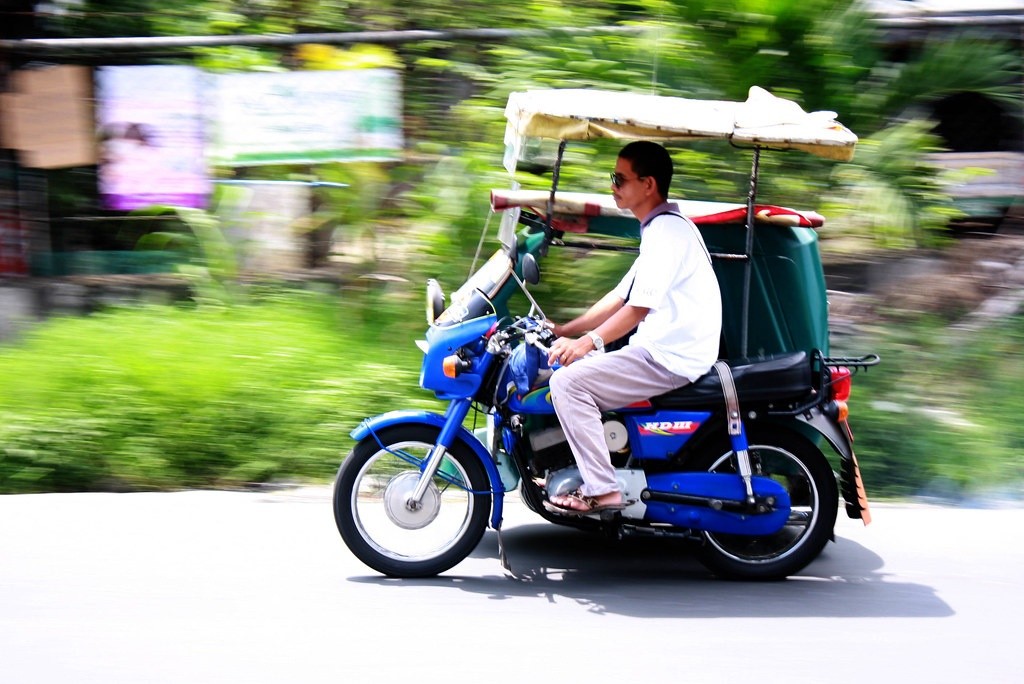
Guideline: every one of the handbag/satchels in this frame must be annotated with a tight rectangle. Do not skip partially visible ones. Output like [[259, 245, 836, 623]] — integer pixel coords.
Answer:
[[607, 327, 637, 352]]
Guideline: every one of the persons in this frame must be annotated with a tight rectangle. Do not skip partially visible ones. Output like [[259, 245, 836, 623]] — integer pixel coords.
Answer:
[[521, 138, 724, 516]]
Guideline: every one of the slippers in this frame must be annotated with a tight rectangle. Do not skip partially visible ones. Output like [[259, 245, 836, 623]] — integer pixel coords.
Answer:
[[543, 488, 625, 516]]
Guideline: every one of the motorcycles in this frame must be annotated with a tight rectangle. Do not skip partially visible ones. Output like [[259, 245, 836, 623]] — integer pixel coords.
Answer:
[[333, 225, 880, 582]]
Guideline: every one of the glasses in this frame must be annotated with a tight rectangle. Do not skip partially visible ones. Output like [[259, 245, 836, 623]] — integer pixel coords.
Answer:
[[611, 170, 647, 189]]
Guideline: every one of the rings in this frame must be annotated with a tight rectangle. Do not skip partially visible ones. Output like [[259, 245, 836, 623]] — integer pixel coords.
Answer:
[[563, 354, 568, 360]]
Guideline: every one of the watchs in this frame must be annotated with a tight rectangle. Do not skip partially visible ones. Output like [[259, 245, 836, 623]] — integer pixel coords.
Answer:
[[587, 331, 605, 351]]
